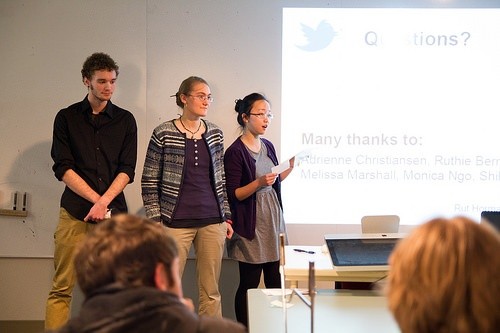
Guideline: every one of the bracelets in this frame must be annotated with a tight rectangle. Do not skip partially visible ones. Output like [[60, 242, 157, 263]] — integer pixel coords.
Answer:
[[225, 219, 233, 224]]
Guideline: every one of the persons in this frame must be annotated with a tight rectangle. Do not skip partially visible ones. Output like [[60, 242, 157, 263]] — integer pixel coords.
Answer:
[[387, 216, 500, 333], [224, 93, 295, 333], [45, 52, 138, 333], [141, 76, 234, 319], [57, 213, 247, 333]]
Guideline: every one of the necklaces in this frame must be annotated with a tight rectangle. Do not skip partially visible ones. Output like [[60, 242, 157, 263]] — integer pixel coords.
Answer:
[[180, 116, 202, 139]]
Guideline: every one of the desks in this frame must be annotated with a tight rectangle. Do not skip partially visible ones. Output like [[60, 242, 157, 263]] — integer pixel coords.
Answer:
[[246, 288, 400, 333], [282, 233, 409, 292]]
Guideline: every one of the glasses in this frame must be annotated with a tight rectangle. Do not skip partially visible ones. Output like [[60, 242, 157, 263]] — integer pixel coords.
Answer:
[[189, 95, 213, 103], [251, 112, 273, 119]]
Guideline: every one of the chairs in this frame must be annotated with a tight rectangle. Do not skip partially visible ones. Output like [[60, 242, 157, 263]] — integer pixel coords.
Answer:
[[362, 216, 400, 231]]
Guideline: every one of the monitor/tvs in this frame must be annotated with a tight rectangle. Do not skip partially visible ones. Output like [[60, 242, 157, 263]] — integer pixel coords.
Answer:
[[323, 232, 408, 271]]
